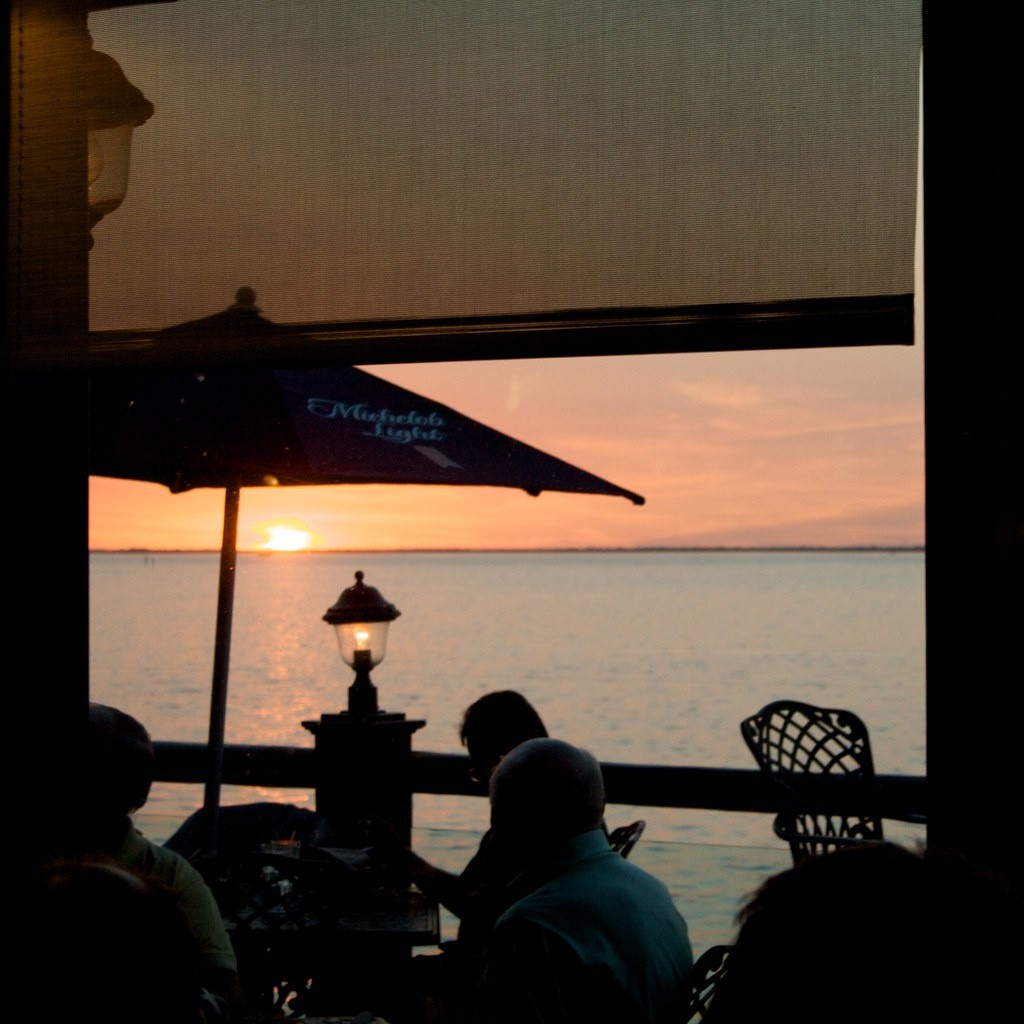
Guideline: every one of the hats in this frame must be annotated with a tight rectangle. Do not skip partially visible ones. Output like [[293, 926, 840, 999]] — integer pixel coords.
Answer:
[[491, 737, 605, 832], [90, 704, 153, 807]]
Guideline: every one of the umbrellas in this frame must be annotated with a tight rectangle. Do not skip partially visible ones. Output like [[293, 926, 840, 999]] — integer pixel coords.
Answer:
[[0, 286, 645, 851]]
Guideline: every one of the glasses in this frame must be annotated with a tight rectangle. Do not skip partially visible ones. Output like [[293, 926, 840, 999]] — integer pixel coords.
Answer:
[[469, 767, 482, 782]]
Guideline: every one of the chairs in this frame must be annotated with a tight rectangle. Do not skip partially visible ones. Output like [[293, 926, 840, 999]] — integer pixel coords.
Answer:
[[739, 699, 886, 866], [683, 944, 737, 1024], [607, 819, 646, 858]]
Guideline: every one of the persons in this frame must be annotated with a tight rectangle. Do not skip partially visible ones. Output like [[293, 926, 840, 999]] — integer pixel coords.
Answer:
[[0, 704, 239, 1024], [365, 691, 547, 1024], [472, 739, 693, 1024], [700, 842, 975, 1024]]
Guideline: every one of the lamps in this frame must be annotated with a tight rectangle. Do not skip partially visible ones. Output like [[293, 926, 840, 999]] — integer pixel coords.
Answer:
[[321, 570, 407, 721]]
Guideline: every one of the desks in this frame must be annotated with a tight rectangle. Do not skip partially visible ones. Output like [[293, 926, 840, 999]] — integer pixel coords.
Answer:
[[238, 891, 440, 1024]]
